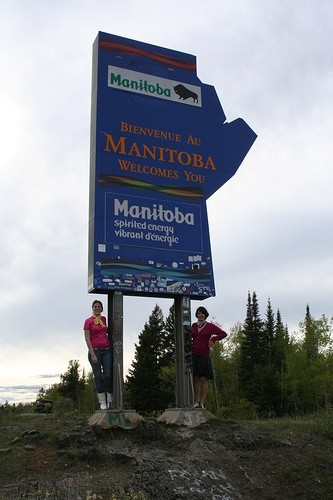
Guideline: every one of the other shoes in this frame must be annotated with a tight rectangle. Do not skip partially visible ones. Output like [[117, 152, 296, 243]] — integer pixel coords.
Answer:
[[195, 403, 204, 407]]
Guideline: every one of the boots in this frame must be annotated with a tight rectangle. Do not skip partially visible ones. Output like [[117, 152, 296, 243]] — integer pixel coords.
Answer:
[[97, 392, 112, 409]]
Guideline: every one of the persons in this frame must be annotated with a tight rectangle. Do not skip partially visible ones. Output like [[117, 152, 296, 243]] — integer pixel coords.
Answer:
[[83, 300, 114, 411], [191, 306, 227, 408]]
